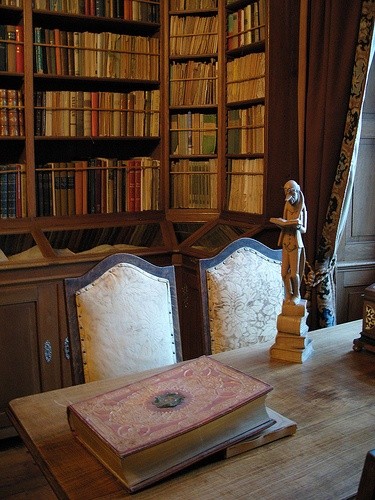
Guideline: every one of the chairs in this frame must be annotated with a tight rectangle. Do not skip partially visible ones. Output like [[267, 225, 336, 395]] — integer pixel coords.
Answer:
[[62, 253, 180, 385], [198, 238, 285, 356]]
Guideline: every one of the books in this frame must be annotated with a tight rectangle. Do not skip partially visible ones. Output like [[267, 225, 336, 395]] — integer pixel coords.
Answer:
[[0, 0, 270, 219], [65, 355, 297, 493]]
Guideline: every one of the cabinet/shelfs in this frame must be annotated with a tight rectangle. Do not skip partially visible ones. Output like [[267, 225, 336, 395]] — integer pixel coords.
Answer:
[[1, 0, 163, 441], [163, 0, 296, 361]]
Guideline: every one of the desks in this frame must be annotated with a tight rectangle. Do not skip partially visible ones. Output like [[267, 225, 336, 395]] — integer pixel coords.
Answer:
[[7, 318, 375, 500]]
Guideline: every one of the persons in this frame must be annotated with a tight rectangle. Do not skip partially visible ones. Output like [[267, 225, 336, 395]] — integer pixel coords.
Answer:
[[278, 181, 307, 303]]
